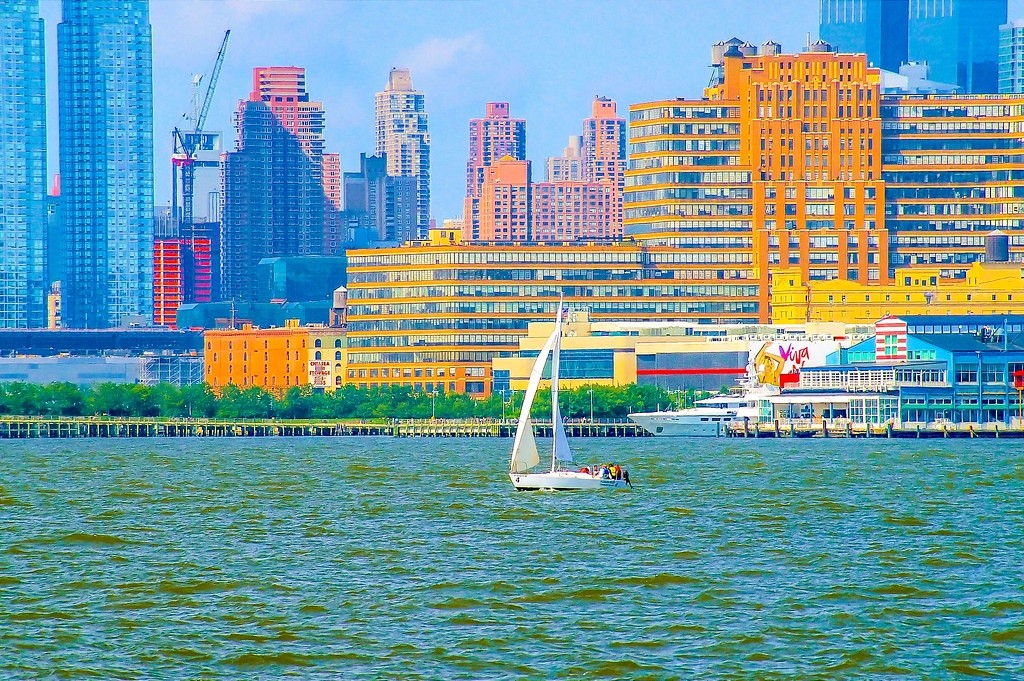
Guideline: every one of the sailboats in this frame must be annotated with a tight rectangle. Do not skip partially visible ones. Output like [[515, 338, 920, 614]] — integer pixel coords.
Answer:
[[509, 289, 632, 490]]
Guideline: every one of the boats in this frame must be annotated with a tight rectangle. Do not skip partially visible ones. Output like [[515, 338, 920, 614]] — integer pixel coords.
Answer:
[[626, 376, 761, 438]]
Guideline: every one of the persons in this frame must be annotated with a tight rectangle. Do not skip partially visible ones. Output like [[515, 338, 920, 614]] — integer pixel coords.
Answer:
[[578, 461, 633, 489]]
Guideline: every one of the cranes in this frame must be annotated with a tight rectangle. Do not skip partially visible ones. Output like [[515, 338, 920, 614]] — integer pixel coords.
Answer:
[[171, 28, 232, 301]]
